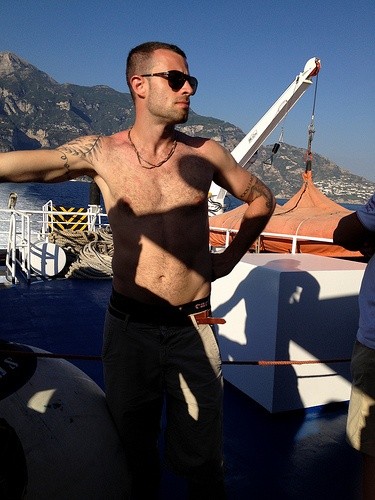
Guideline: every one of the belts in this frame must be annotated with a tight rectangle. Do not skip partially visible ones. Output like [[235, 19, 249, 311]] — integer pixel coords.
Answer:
[[107, 303, 226, 326]]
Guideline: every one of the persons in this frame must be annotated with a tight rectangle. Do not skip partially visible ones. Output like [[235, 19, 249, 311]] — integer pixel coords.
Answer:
[[332, 192, 375, 500], [0, 42, 276, 500]]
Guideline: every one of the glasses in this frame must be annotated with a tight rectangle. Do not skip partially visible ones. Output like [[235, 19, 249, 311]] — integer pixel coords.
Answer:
[[141, 70, 197, 94]]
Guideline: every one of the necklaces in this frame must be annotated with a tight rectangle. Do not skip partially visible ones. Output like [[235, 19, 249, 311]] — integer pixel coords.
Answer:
[[128, 128, 177, 170]]
